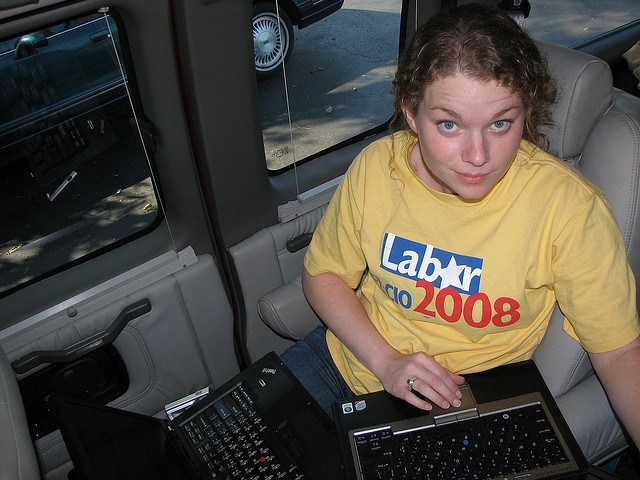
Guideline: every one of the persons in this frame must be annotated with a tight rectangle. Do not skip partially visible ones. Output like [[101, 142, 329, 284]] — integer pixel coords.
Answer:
[[278, 3, 640, 450]]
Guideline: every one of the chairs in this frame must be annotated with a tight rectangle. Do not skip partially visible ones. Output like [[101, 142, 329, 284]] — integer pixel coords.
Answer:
[[259, 36, 639, 477]]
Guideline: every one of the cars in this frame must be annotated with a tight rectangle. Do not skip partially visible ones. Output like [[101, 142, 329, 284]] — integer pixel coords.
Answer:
[[0, 0, 344, 151]]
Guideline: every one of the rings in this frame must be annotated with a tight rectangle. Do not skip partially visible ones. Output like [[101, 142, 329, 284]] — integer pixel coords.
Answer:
[[406, 376, 419, 392]]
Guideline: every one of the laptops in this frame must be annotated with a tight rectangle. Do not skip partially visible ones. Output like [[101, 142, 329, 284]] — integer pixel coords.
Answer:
[[331, 359, 624, 480], [49, 351, 346, 480]]
